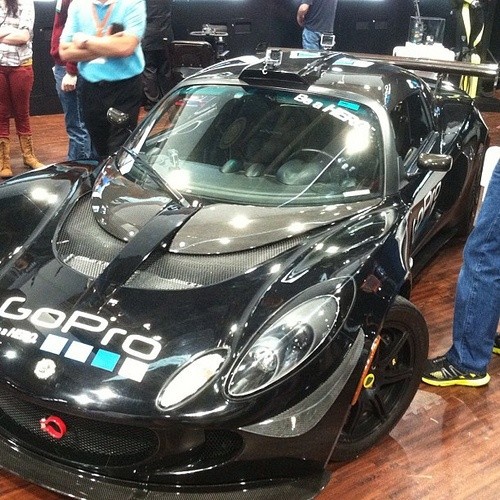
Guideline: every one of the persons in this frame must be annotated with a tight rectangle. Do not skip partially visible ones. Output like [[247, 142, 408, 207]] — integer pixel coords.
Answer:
[[49, 0, 175, 158], [421, 157, 500, 385], [296, 0, 338, 51], [0, 0, 46, 179]]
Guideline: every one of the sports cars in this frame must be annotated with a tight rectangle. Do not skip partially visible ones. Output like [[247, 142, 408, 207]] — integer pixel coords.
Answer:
[[0, 34, 500, 500]]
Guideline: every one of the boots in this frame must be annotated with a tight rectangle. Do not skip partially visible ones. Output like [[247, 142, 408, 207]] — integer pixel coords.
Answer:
[[17, 127, 45, 169], [0, 138, 13, 177]]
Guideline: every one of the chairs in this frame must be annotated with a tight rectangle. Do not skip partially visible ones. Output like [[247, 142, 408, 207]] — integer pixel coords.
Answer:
[[235, 105, 382, 173]]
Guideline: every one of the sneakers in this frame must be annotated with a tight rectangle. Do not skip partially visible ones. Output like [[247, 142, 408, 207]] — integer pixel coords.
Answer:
[[420, 352, 490, 386], [492, 335, 500, 354]]
